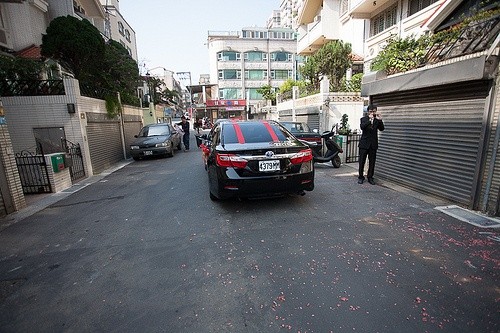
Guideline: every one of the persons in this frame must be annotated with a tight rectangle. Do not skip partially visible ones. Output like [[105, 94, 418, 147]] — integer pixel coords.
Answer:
[[174, 117, 190, 151], [358, 105, 385, 185]]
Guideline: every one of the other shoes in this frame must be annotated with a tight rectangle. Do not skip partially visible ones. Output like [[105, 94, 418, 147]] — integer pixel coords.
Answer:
[[184, 149, 189, 151]]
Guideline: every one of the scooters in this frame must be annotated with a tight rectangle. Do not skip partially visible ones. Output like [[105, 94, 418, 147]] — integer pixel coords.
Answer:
[[297, 124, 343, 168]]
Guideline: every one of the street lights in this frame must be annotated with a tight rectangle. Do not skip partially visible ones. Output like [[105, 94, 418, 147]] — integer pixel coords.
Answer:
[[146, 72, 151, 102]]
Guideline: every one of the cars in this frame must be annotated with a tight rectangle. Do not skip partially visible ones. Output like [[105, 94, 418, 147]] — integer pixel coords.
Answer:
[[129, 123, 182, 160], [195, 120, 321, 201]]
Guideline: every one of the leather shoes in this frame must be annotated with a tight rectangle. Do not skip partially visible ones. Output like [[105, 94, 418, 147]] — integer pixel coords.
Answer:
[[368, 179, 375, 185], [358, 179, 363, 184]]
